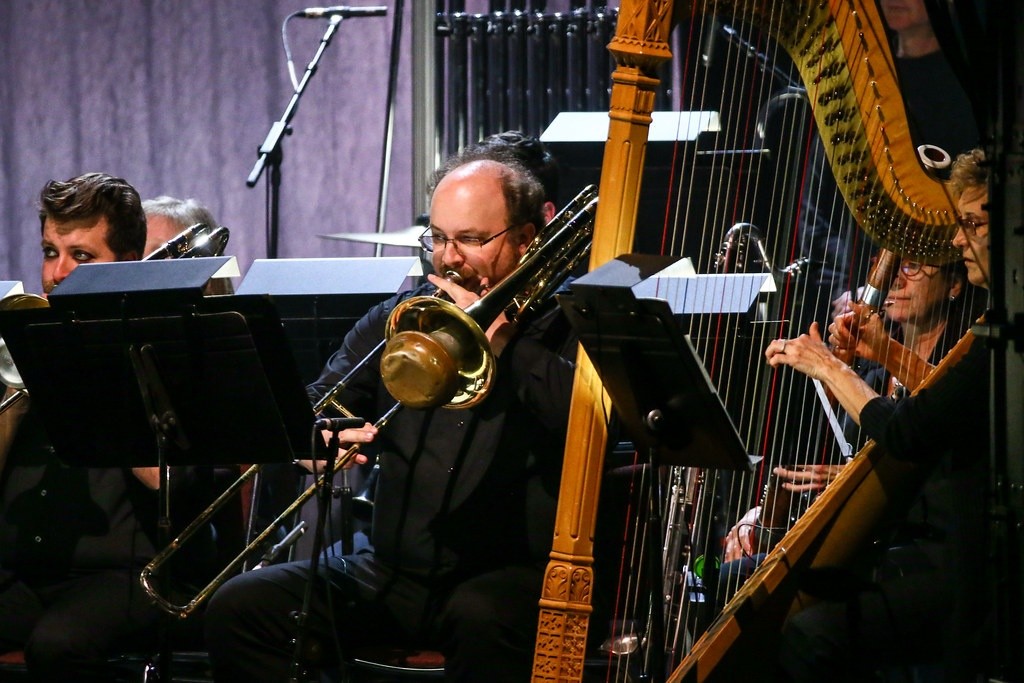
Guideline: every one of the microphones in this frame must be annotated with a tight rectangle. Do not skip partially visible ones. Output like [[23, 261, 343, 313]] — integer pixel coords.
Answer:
[[254, 521, 307, 572], [296, 6, 387, 19]]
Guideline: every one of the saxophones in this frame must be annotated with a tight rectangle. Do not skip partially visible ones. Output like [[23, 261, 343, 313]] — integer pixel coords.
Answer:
[[598, 220, 812, 682]]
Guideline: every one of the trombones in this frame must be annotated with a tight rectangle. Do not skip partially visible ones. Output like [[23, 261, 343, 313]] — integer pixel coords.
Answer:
[[0, 222, 231, 415], [139, 185, 600, 618]]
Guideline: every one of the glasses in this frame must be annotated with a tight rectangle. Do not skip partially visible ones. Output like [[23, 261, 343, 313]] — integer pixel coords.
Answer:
[[900, 258, 926, 278], [961, 218, 990, 237], [417, 225, 525, 258]]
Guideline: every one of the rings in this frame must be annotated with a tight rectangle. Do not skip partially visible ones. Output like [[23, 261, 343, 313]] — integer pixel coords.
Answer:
[[780, 342, 787, 353]]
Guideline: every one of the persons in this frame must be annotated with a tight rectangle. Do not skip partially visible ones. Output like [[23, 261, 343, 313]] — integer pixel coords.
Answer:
[[138, 193, 241, 298], [0, 174, 214, 682], [766, 146, 995, 682], [725, 253, 969, 560], [451, 129, 567, 228], [198, 160, 579, 682]]
